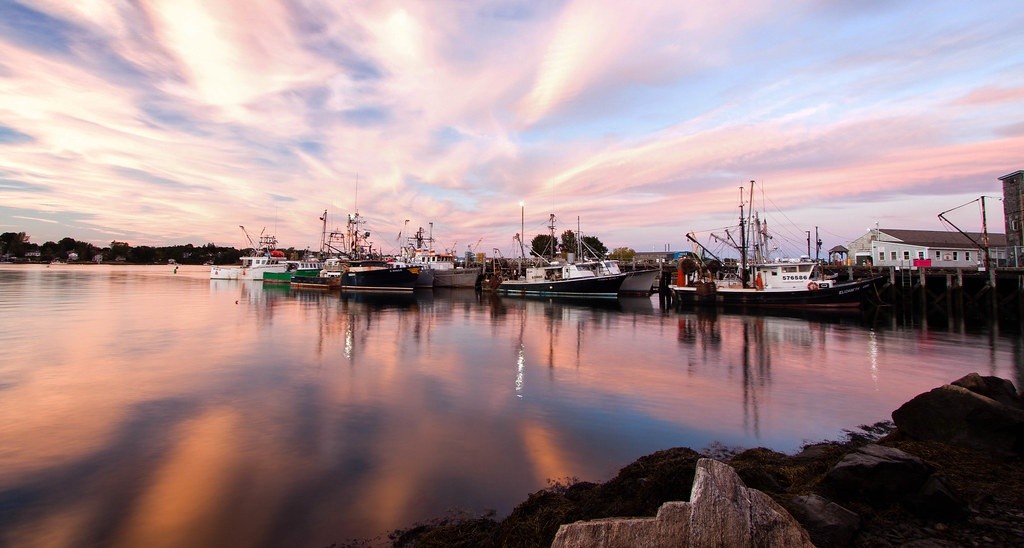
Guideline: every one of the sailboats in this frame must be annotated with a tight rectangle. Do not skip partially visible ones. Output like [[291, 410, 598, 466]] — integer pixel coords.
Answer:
[[667, 180, 884, 315], [496, 213, 628, 301], [571, 216, 661, 297]]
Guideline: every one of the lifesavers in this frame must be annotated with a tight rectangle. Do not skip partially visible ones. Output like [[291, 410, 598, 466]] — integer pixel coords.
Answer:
[[807, 282, 819, 291]]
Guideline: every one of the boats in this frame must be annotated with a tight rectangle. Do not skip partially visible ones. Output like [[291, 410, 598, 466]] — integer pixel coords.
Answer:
[[387, 223, 481, 288], [339, 260, 423, 294], [286, 246, 324, 277], [210, 225, 289, 280]]
